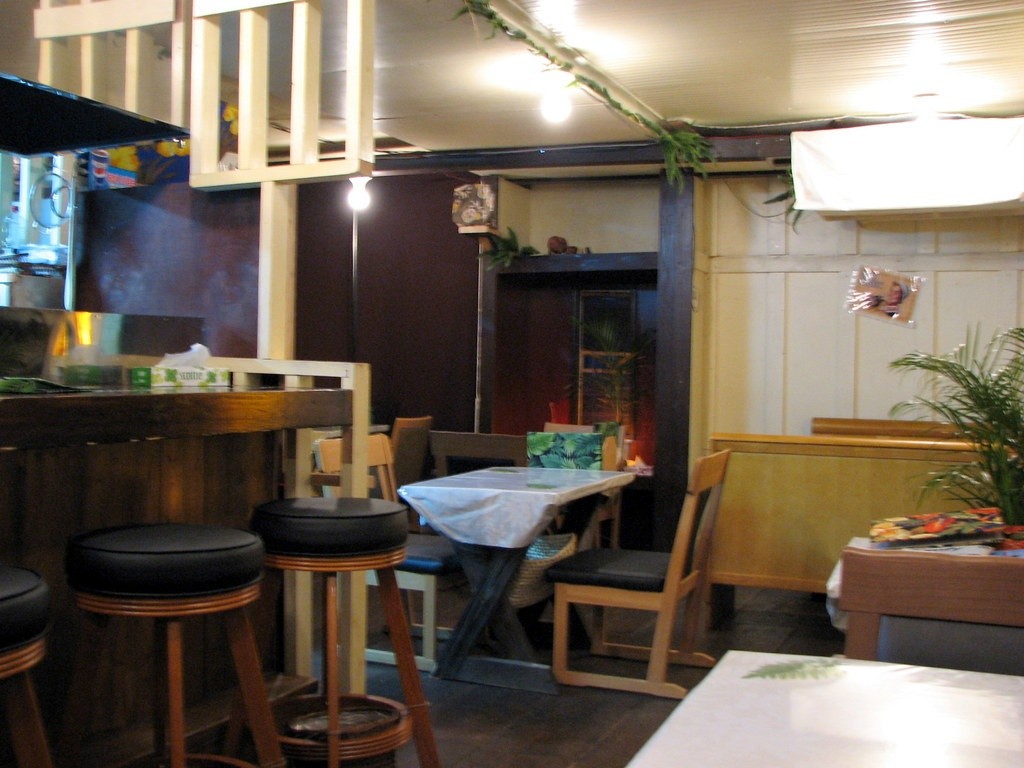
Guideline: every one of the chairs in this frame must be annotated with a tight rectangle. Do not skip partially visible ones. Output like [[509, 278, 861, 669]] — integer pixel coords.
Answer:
[[544, 446, 732, 701], [314, 434, 456, 675], [841, 547, 1024, 676], [368, 414, 434, 521]]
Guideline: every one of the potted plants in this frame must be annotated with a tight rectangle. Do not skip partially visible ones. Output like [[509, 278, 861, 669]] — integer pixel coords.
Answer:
[[890, 325, 1024, 548]]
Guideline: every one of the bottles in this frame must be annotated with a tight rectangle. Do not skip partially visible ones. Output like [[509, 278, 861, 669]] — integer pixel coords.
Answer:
[[4, 206, 21, 247]]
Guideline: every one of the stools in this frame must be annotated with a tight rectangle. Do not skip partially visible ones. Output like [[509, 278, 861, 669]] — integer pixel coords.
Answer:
[[57, 523, 286, 768], [0, 565, 53, 768], [222, 496, 442, 768]]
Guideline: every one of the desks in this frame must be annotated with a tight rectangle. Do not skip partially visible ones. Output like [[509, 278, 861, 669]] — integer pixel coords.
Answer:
[[624, 650, 1024, 768], [827, 537, 1024, 632], [396, 466, 634, 697]]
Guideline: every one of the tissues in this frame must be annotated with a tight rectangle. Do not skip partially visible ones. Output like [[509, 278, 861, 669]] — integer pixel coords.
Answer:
[[126, 343, 229, 387]]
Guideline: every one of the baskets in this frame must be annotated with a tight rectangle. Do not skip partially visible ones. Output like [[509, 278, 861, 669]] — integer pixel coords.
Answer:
[[503, 526, 577, 609]]
